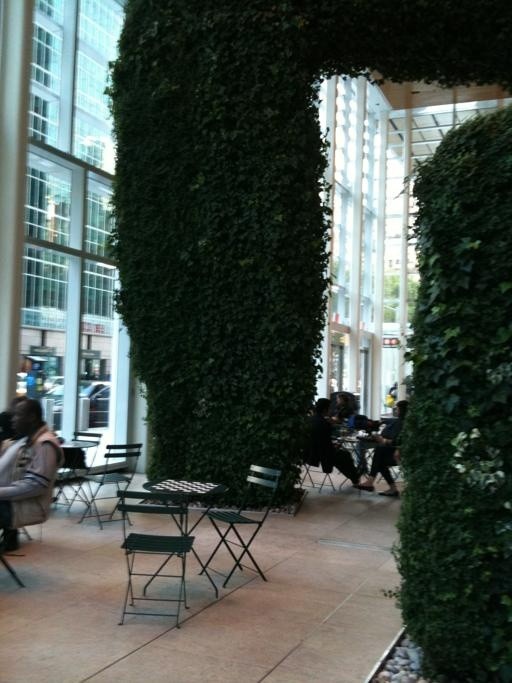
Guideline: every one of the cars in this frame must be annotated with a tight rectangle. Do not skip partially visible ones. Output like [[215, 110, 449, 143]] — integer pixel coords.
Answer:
[[387, 373, 416, 398], [17, 368, 109, 434]]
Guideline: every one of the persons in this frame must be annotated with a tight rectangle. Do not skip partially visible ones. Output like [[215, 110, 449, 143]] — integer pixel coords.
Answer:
[[0, 395, 30, 555], [352, 398, 409, 497], [303, 395, 360, 483], [328, 391, 357, 422], [1, 398, 65, 532]]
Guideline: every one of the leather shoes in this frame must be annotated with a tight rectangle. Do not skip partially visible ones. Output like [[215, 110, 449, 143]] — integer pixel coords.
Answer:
[[353, 485, 399, 497]]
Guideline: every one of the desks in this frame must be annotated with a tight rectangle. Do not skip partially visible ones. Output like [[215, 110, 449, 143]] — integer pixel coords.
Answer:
[[336, 435, 382, 492]]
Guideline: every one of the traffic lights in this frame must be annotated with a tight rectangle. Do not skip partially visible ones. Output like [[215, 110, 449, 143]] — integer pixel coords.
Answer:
[[383, 336, 400, 347]]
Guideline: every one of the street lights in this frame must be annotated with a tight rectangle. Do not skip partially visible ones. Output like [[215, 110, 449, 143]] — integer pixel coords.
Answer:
[[342, 291, 400, 389]]
